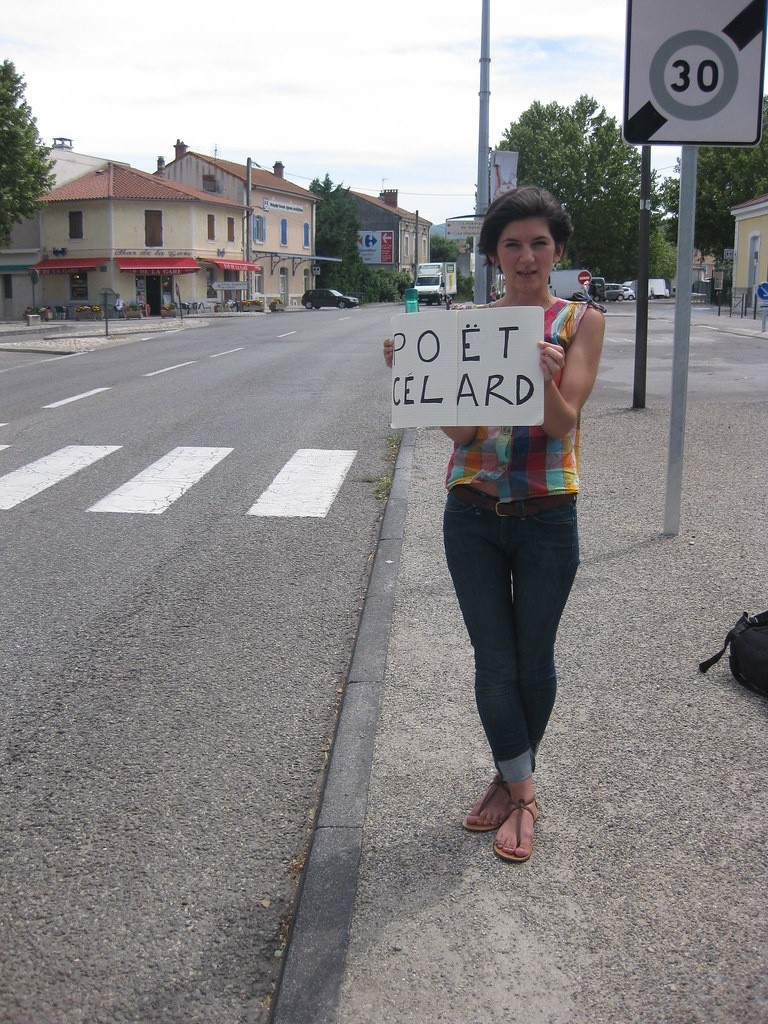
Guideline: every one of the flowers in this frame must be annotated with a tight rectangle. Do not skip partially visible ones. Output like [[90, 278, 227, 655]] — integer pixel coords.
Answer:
[[162, 302, 175, 311], [75, 305, 102, 313], [240, 299, 265, 306], [270, 298, 284, 305], [24, 307, 50, 315]]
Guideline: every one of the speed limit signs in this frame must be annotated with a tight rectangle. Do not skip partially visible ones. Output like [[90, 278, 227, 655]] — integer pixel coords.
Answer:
[[623, 0, 765, 148]]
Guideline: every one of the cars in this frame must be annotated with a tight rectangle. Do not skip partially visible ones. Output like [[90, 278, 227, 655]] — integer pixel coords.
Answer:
[[621, 286, 635, 300]]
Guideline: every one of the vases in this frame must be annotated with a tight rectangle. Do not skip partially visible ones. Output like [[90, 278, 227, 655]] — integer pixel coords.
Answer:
[[160, 309, 176, 319], [269, 304, 287, 312], [23, 312, 52, 322], [75, 311, 104, 321], [242, 304, 264, 312]]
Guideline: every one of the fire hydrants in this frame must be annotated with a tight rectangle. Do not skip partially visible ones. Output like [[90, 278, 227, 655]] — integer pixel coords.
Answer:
[[445, 294, 452, 310]]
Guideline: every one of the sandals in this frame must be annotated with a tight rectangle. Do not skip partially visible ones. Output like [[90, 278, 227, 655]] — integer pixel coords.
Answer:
[[493, 796, 540, 861], [461, 773, 512, 832]]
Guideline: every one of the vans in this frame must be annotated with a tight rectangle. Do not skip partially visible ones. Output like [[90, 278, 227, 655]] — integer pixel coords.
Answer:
[[590, 276, 606, 302]]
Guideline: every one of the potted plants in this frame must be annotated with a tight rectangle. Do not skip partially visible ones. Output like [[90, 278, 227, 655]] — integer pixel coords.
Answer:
[[125, 303, 143, 319]]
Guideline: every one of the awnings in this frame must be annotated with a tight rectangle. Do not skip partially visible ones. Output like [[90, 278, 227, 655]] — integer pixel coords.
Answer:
[[198, 258, 262, 272], [252, 251, 342, 275], [29, 258, 110, 274], [113, 258, 202, 274]]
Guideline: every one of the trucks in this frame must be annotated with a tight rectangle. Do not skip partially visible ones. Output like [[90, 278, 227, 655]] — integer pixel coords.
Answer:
[[413, 262, 457, 307], [547, 269, 590, 300], [623, 278, 666, 299]]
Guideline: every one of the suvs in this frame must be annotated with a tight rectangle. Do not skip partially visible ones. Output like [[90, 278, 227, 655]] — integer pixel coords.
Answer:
[[604, 283, 625, 302], [301, 289, 360, 309]]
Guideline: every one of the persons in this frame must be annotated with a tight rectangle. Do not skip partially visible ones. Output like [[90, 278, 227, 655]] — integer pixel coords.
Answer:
[[113, 294, 123, 317], [490, 289, 496, 301], [588, 280, 598, 301], [383, 186, 606, 862]]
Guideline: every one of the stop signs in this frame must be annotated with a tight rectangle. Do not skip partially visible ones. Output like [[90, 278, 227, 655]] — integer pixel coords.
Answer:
[[578, 270, 592, 285]]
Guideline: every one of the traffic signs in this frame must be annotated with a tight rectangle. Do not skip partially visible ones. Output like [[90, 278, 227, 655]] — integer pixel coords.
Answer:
[[211, 280, 247, 291]]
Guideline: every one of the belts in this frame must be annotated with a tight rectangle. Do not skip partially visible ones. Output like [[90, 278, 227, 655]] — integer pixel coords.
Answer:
[[451, 484, 577, 518]]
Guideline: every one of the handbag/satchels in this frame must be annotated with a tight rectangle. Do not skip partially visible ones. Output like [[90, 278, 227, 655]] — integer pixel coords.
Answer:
[[699, 612, 768, 697]]
